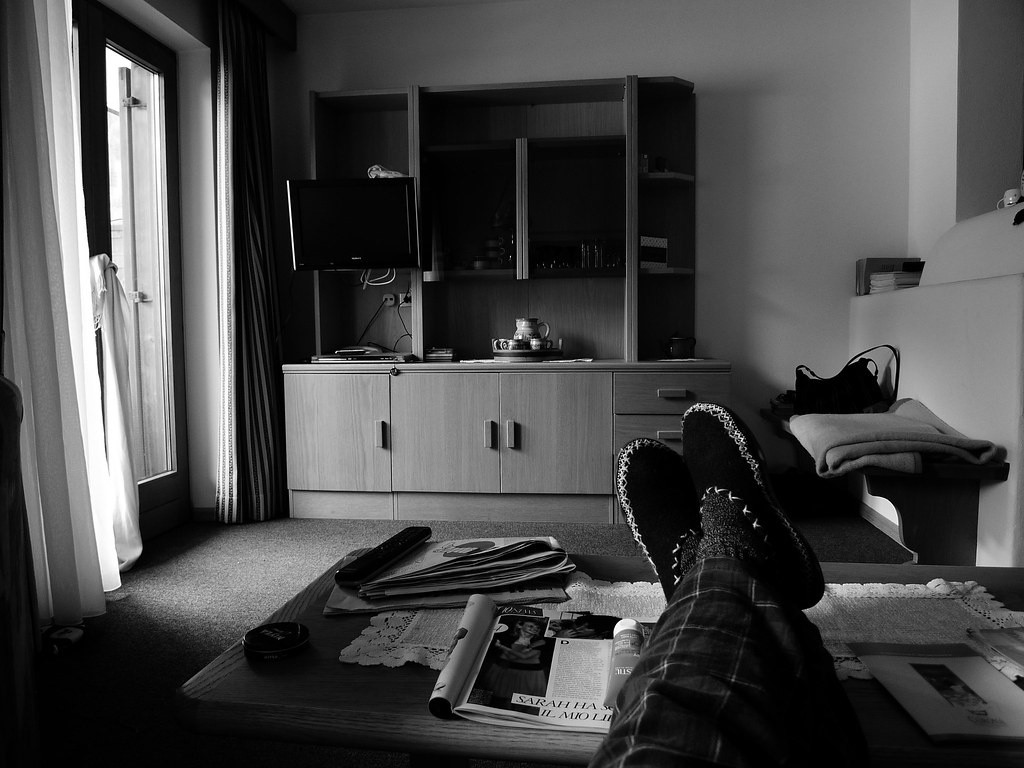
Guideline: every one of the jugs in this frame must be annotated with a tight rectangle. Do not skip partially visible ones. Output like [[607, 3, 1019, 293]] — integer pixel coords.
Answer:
[[514, 317, 550, 350], [667, 337, 696, 358]]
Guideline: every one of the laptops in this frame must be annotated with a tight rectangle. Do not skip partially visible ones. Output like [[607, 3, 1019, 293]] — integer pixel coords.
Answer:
[[311, 354, 413, 364]]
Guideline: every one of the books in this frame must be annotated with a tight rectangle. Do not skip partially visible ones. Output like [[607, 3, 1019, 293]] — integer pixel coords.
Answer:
[[427, 594, 623, 735], [425, 348, 453, 361], [843, 642, 1023, 741]]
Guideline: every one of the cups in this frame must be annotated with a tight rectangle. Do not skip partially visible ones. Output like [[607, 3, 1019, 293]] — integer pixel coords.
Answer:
[[530, 338, 553, 351], [494, 339, 525, 350], [997, 189, 1020, 209]]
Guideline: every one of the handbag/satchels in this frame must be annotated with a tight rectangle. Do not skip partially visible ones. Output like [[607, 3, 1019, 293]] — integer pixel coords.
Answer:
[[795, 344, 899, 414]]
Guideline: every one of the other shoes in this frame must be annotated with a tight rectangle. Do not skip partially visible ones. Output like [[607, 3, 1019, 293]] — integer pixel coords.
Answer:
[[680, 403, 825, 610], [613, 437, 706, 604]]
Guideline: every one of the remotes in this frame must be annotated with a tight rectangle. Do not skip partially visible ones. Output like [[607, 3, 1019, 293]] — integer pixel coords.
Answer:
[[336, 526, 433, 589], [335, 350, 365, 354]]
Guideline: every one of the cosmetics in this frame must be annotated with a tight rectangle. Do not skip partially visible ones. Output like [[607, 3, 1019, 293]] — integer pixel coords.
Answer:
[[244, 622, 310, 663], [602, 618, 645, 708]]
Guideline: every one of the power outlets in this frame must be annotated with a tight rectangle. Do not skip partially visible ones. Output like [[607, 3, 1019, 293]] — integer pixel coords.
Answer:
[[383, 294, 396, 307], [399, 293, 411, 307]]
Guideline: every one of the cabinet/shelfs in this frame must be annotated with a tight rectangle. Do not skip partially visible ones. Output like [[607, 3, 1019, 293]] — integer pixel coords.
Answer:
[[278, 74, 736, 527]]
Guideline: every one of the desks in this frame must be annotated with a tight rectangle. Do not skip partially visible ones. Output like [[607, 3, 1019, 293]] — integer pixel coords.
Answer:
[[764, 406, 980, 566], [172, 545, 1024, 768]]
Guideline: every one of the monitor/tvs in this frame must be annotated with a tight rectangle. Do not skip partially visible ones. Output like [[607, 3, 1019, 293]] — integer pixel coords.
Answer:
[[287, 177, 420, 272]]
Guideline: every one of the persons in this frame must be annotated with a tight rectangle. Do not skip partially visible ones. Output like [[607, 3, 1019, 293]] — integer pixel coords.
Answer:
[[587, 399, 874, 768], [489, 618, 546, 701]]
